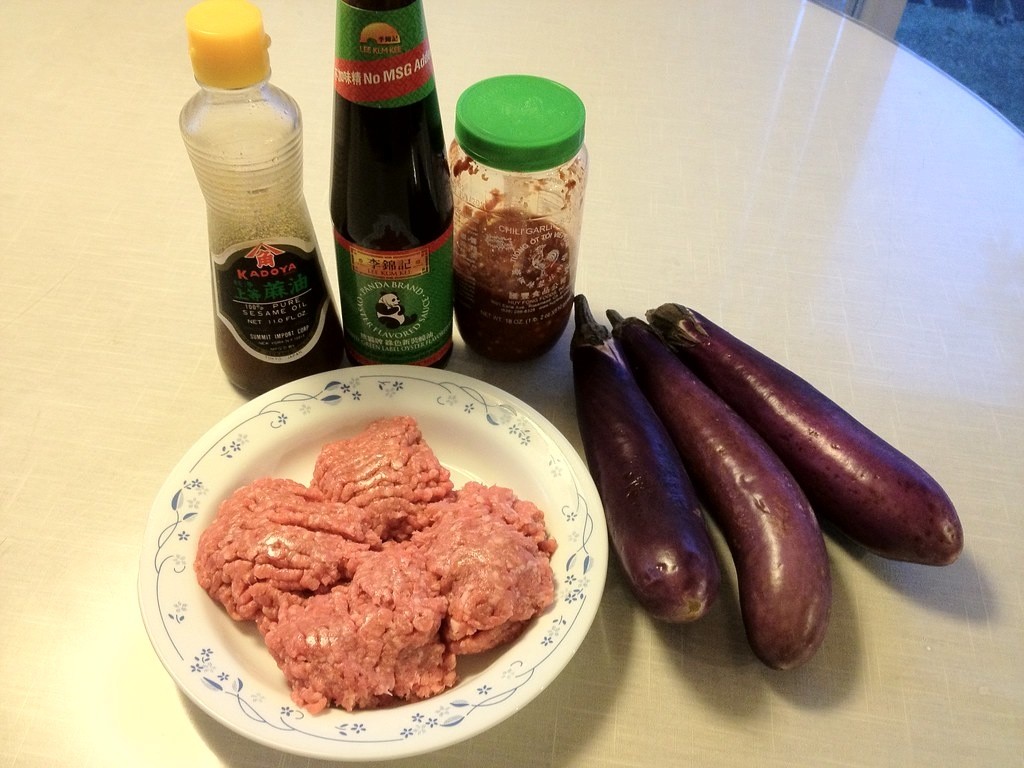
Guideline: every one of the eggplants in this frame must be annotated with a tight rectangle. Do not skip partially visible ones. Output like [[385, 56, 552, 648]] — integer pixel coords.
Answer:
[[573, 292, 965, 672]]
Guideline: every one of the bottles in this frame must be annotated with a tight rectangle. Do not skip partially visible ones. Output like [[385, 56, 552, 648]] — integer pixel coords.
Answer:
[[448, 76, 589, 362], [179, 1, 349, 401], [330, 0, 454, 368]]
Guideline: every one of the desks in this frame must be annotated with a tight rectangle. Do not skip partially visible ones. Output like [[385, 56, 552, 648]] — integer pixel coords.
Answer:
[[0, 1, 1023, 766]]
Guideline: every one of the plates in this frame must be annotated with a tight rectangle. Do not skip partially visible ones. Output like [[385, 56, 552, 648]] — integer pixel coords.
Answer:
[[138, 366, 610, 761]]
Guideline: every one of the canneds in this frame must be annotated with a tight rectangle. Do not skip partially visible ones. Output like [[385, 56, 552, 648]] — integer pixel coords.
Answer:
[[447, 74, 588, 364]]
